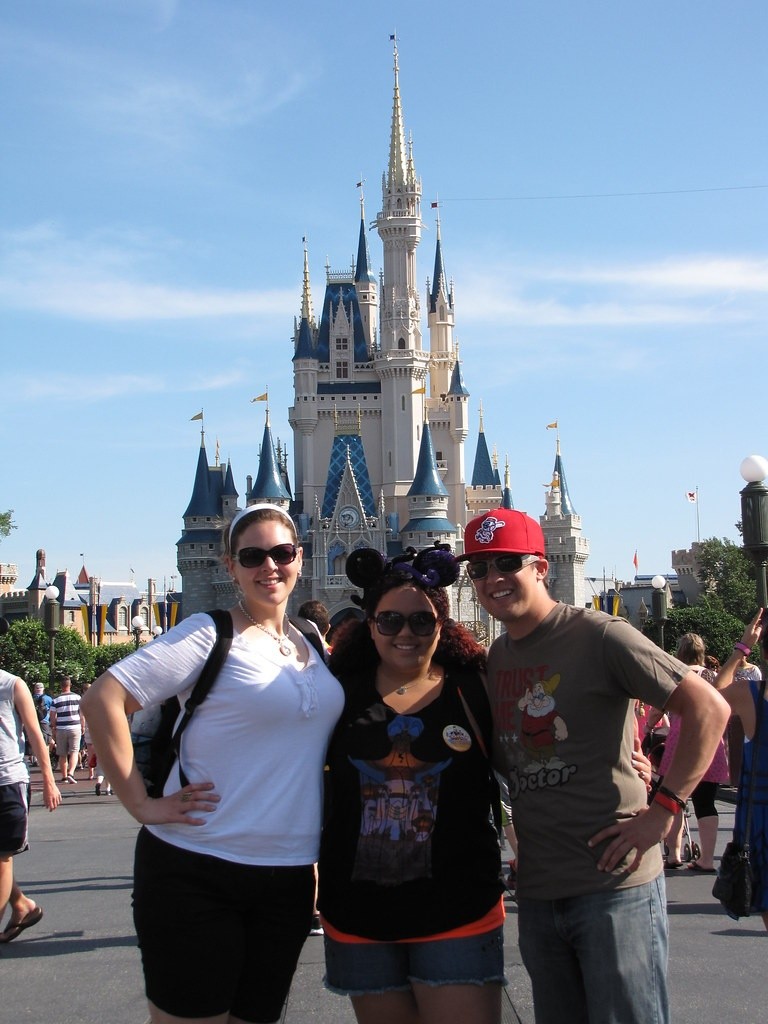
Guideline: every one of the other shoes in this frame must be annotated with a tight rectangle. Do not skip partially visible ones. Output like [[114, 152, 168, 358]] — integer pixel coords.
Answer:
[[106, 790, 114, 795], [96, 783, 102, 795]]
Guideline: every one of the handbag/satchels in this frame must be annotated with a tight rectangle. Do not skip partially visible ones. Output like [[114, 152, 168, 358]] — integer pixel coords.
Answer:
[[712, 842, 758, 918]]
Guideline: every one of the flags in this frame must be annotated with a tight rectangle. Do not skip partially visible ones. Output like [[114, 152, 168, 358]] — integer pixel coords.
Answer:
[[633, 554, 638, 571], [686, 492, 696, 504]]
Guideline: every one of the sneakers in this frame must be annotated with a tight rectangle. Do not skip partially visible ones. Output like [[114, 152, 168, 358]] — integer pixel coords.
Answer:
[[67, 774, 79, 783], [310, 913, 325, 936], [60, 777, 69, 783]]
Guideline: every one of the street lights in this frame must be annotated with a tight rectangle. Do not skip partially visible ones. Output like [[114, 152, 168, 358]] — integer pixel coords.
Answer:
[[650, 575, 668, 651], [738, 454, 768, 618], [45, 585, 62, 692], [131, 616, 144, 650], [153, 625, 163, 644]]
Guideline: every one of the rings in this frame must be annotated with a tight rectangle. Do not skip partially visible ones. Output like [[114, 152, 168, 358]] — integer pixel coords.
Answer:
[[178, 792, 192, 800]]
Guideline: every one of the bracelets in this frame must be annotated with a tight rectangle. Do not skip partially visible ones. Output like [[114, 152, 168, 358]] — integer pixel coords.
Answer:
[[646, 722, 653, 730], [734, 642, 750, 656], [652, 785, 688, 815]]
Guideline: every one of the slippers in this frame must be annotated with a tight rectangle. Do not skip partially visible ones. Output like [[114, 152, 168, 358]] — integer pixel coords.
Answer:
[[663, 858, 684, 868], [0, 908, 44, 941], [688, 861, 718, 874]]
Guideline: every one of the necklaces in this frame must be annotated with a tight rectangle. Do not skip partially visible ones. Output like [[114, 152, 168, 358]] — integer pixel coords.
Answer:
[[238, 600, 292, 656], [377, 667, 433, 695]]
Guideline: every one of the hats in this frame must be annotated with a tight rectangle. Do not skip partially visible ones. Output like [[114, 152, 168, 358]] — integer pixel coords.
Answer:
[[455, 507, 545, 562]]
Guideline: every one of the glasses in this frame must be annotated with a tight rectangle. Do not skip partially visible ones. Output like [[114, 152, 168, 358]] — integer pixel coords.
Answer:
[[233, 544, 299, 568], [467, 553, 540, 579], [372, 611, 440, 636]]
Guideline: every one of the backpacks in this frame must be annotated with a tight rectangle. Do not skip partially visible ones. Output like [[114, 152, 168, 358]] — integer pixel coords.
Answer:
[[131, 609, 325, 797], [33, 694, 48, 721]]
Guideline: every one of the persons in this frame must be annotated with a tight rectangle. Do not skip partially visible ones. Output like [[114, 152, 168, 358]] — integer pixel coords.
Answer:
[[77, 683, 113, 797], [490, 771, 520, 892], [293, 541, 652, 1024], [78, 502, 344, 1024], [28, 682, 53, 767], [0, 668, 63, 944], [456, 507, 733, 1024], [631, 633, 730, 876], [716, 608, 768, 929], [51, 676, 85, 784]]
